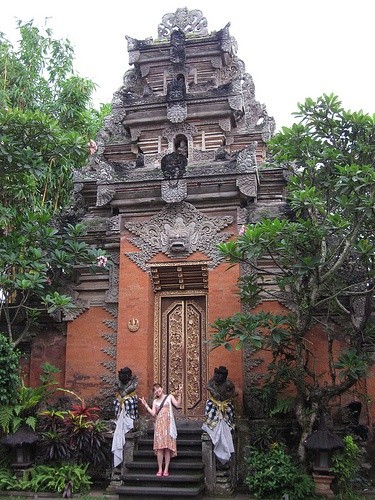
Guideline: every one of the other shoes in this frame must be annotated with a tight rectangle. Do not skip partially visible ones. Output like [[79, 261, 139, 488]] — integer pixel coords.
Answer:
[[156, 471, 169, 477]]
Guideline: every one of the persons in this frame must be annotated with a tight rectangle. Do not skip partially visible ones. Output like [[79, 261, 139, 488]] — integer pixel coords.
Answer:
[[140, 382, 184, 478]]
